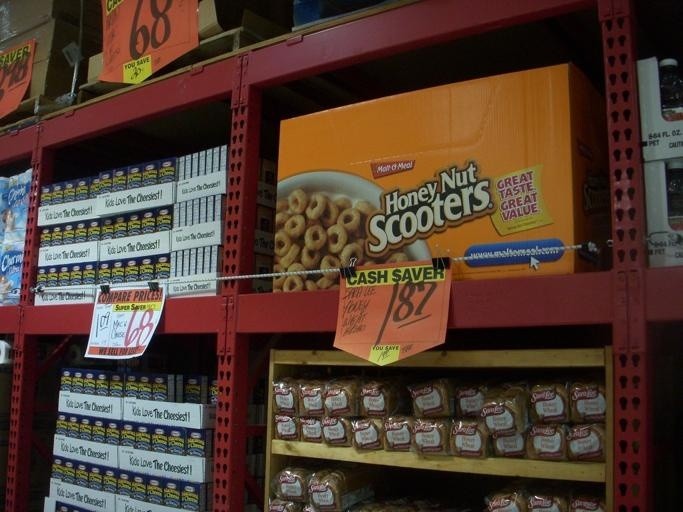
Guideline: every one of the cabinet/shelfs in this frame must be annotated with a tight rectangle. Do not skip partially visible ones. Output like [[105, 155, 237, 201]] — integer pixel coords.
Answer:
[[0, 0, 682, 512]]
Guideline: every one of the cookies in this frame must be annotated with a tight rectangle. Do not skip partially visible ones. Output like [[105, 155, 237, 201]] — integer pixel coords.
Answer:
[[273, 188, 408, 294]]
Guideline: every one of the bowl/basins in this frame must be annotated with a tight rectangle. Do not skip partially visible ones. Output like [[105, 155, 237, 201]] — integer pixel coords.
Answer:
[[277, 169, 434, 261]]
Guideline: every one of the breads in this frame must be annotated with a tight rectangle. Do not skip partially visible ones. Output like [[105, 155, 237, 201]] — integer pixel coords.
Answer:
[[270, 373, 609, 512]]
